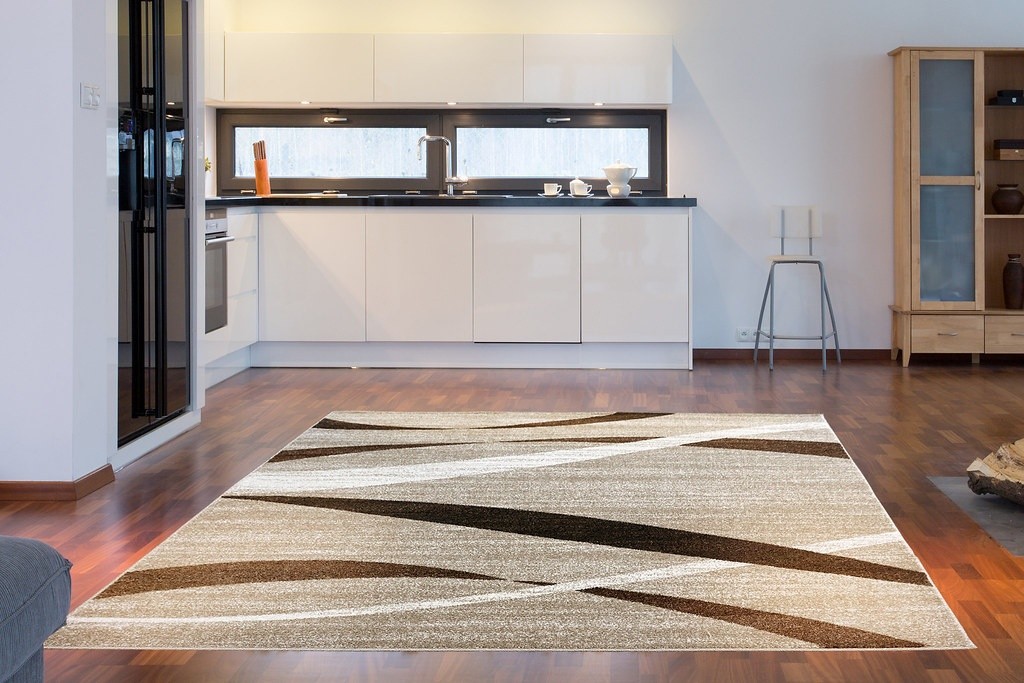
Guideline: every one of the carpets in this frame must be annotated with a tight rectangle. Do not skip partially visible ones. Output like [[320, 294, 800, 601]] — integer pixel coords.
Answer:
[[50, 410, 974, 652]]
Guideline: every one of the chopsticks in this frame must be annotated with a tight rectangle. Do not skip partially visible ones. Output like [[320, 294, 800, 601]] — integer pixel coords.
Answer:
[[253, 140, 266, 159]]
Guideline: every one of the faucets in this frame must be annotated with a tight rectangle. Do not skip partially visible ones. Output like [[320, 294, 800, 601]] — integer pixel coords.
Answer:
[[416, 135, 466, 195]]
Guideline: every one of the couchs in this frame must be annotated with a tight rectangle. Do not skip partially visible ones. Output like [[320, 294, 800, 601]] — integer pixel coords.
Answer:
[[1, 534, 72, 683]]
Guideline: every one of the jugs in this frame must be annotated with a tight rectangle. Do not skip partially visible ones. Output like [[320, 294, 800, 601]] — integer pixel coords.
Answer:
[[570, 178, 584, 194], [603, 160, 637, 197]]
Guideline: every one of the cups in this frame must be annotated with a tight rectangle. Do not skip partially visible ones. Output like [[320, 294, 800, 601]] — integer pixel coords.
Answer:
[[254, 160, 270, 195], [575, 183, 592, 194], [544, 183, 562, 195]]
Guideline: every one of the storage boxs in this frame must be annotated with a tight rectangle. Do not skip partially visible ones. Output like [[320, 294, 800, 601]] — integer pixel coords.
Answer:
[[995, 139, 1024, 149], [993, 149, 1024, 161], [989, 97, 1024, 106]]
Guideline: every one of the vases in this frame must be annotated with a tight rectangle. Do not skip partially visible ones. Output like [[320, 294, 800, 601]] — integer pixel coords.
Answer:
[[1003, 253, 1024, 308], [991, 183, 1024, 214]]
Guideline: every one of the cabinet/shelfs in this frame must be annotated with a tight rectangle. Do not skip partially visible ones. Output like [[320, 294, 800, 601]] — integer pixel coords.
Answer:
[[206, 209, 693, 390], [888, 45, 1024, 368], [205, 30, 674, 105]]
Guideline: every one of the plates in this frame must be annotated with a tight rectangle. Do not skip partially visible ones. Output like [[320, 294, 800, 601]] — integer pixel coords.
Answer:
[[542, 195, 559, 197], [572, 195, 589, 197]]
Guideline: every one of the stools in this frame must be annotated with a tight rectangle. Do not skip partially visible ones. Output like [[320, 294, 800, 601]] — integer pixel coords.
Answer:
[[753, 202, 842, 371]]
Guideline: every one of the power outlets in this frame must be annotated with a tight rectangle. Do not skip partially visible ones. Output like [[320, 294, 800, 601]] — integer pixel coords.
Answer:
[[737, 327, 775, 343]]
